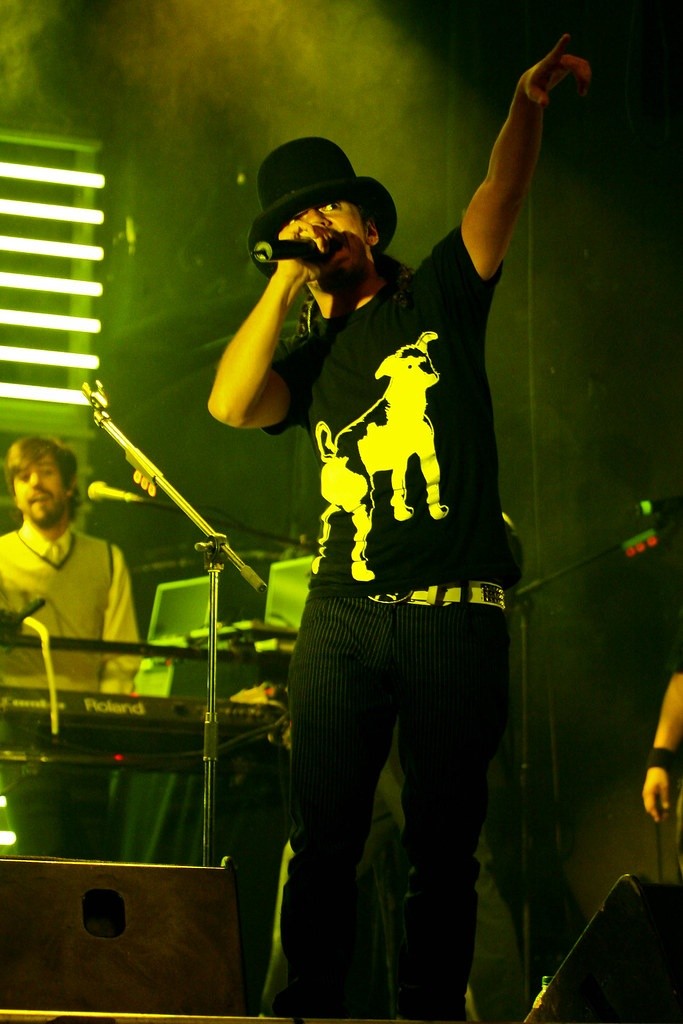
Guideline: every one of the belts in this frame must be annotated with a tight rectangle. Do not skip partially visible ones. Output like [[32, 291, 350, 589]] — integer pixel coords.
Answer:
[[365, 579, 509, 612]]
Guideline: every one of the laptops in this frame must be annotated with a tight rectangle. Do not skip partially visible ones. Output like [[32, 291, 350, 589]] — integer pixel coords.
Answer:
[[264, 555, 317, 654], [148, 573, 218, 644]]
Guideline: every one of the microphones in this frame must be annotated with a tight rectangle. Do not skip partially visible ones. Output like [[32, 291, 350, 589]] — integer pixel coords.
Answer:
[[250, 237, 344, 264], [88, 481, 144, 506], [628, 495, 683, 517]]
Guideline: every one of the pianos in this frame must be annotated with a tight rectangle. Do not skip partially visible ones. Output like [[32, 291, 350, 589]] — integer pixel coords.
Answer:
[[1, 628, 290, 750]]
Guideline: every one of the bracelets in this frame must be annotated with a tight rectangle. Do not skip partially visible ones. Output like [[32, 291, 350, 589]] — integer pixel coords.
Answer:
[[642, 747, 678, 775]]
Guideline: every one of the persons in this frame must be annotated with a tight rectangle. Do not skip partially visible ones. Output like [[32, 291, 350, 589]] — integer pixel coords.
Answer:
[[205, 31, 592, 1022], [0, 434, 144, 862], [642, 627, 683, 878]]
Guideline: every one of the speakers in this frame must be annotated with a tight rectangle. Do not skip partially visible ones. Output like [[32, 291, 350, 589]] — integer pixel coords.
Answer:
[[524, 873, 683, 1024], [0, 855, 250, 1018]]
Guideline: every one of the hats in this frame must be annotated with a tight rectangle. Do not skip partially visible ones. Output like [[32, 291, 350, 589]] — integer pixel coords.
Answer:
[[245, 137, 399, 279]]
